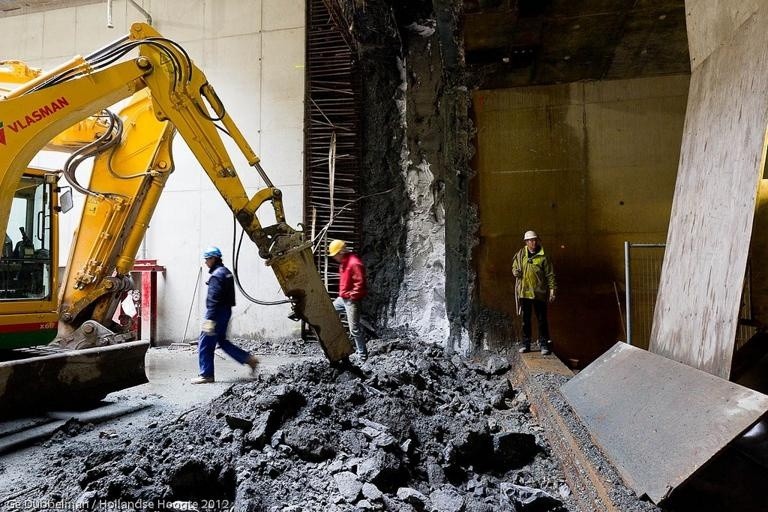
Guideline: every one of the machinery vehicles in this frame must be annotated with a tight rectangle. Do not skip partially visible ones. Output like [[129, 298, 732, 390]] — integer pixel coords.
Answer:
[[1, 20, 355, 425]]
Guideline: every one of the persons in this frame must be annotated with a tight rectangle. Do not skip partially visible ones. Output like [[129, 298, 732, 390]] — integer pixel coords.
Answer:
[[326, 239, 368, 362], [511, 229, 558, 356], [190, 245, 261, 385]]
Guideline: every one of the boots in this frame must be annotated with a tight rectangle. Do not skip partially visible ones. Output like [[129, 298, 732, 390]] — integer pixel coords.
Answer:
[[354, 336, 368, 360]]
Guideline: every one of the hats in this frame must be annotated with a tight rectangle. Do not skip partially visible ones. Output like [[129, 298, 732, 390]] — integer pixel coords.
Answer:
[[524, 230, 538, 240], [201, 246, 222, 258], [327, 240, 347, 257]]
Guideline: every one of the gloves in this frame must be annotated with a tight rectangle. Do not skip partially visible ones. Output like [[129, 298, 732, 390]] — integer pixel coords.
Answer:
[[513, 269, 522, 278], [201, 319, 217, 337], [548, 289, 556, 303]]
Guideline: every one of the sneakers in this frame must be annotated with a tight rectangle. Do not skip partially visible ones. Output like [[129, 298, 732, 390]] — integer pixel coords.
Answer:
[[518, 347, 530, 353], [191, 376, 214, 385], [246, 356, 260, 375], [541, 346, 551, 355]]
